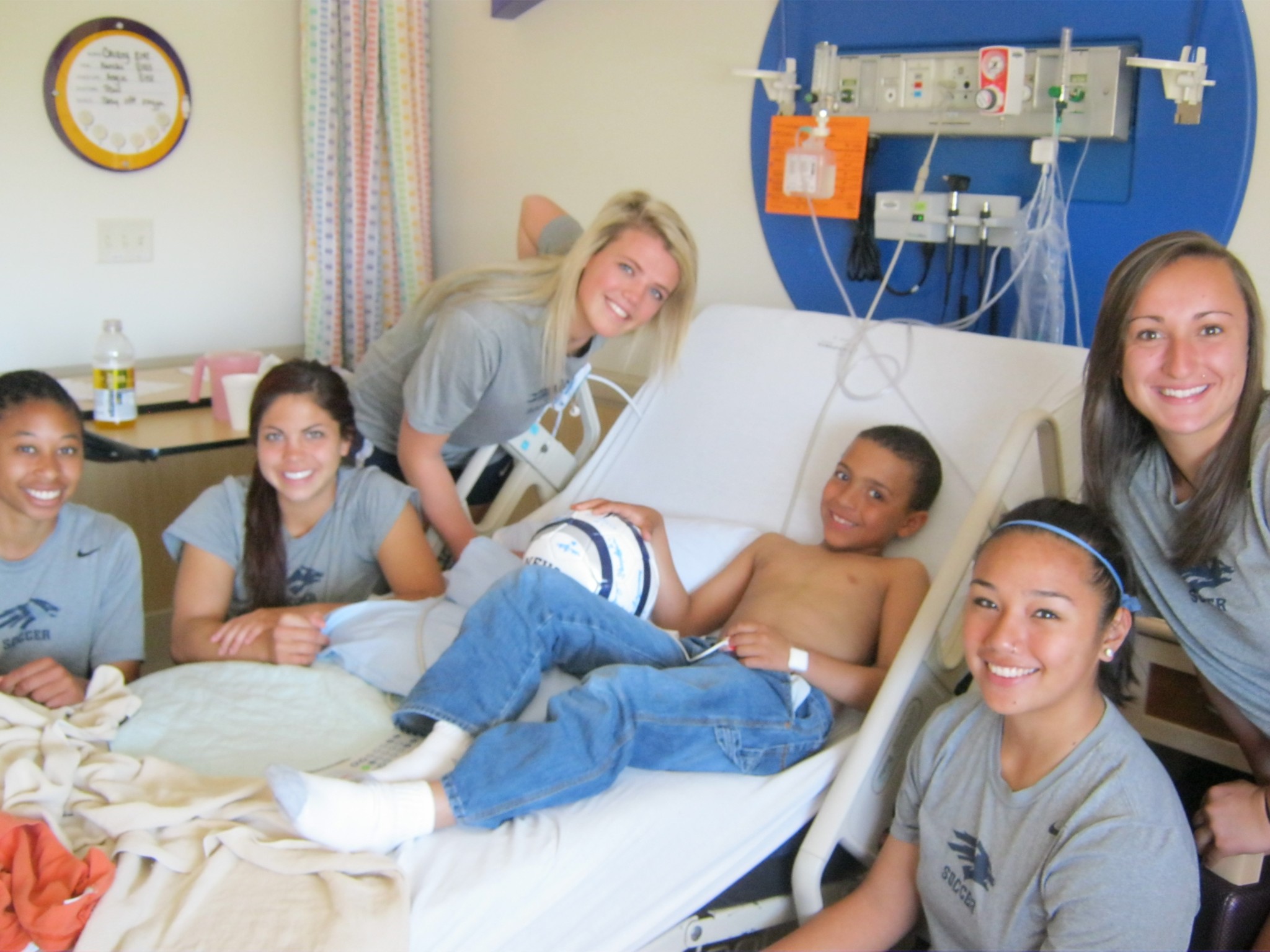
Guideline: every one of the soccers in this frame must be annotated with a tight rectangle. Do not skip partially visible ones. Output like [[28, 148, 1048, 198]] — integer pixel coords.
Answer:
[[520, 507, 660, 619]]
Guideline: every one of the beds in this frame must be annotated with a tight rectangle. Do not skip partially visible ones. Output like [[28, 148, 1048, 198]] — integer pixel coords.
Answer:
[[0, 299, 1095, 952]]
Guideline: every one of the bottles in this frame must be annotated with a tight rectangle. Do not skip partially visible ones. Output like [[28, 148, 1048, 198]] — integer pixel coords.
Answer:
[[93, 319, 140, 434]]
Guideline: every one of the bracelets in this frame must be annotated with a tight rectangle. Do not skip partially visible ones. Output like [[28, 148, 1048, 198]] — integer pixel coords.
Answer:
[[1264, 794, 1270, 823]]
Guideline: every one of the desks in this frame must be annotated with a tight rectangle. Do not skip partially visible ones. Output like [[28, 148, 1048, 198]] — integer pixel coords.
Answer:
[[48, 356, 271, 463]]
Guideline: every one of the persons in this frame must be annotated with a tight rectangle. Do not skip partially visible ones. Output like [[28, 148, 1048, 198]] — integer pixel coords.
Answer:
[[0, 366, 150, 715], [264, 418, 952, 859], [343, 190, 703, 562], [161, 356, 447, 670], [1075, 230, 1270, 952], [753, 495, 1204, 951]]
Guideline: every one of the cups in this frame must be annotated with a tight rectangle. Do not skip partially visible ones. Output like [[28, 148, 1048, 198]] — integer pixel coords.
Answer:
[[191, 348, 263, 433]]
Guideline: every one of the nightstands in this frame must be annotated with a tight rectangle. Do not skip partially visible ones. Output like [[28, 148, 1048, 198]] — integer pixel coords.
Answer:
[[1115, 615, 1250, 782]]
